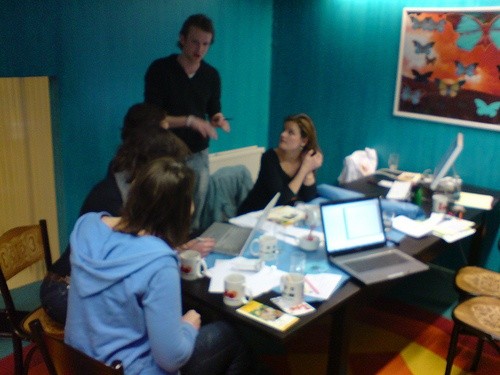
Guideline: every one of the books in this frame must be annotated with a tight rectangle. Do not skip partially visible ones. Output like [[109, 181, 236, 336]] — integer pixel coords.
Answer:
[[236, 301, 299, 332], [271, 296, 315, 317]]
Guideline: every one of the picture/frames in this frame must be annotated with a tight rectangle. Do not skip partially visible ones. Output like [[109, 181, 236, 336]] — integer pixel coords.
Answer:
[[393, 6, 500, 131]]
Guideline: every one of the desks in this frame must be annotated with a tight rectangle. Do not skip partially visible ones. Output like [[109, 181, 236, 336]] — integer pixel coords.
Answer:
[[174, 170, 500, 375]]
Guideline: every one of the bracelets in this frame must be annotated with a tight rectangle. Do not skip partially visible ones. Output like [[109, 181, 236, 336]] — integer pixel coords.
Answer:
[[188, 114, 193, 128]]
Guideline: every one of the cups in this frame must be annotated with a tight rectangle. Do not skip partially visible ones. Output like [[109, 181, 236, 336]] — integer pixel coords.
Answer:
[[432, 194, 448, 214], [281, 274, 305, 304], [179, 250, 207, 280], [382, 210, 395, 232], [223, 273, 253, 307], [250, 235, 277, 262], [298, 235, 320, 252]]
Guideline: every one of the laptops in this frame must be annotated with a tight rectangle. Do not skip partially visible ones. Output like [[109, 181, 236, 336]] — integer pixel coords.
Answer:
[[320, 194, 429, 285], [199, 192, 281, 256]]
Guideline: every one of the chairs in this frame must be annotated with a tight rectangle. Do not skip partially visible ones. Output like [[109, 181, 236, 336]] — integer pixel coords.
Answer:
[[0, 220, 125, 375], [444, 265, 500, 375], [198, 164, 254, 232]]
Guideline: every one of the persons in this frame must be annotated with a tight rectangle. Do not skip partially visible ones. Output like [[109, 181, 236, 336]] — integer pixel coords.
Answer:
[[38, 126, 191, 332], [234, 114, 323, 216], [63, 158, 257, 375], [121, 103, 169, 140], [144, 14, 230, 234]]
